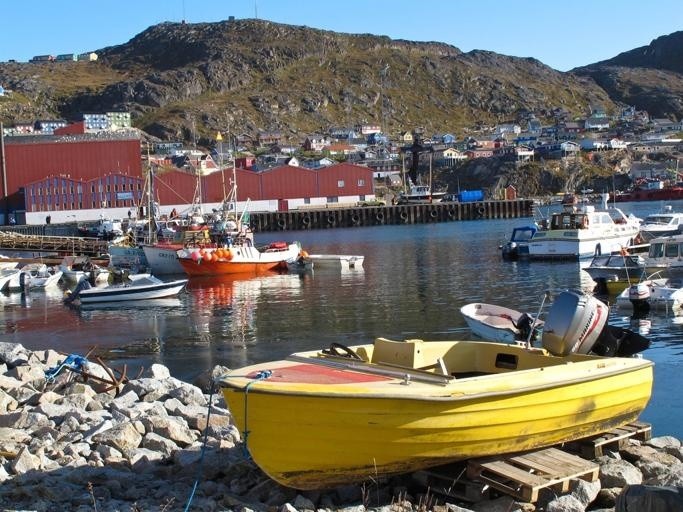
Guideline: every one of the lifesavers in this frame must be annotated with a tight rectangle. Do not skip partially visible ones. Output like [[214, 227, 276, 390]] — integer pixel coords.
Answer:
[[582, 215, 587, 228]]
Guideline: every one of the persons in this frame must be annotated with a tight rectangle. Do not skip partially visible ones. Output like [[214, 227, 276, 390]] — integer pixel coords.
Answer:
[[169, 207, 176, 219], [390, 195, 398, 206]]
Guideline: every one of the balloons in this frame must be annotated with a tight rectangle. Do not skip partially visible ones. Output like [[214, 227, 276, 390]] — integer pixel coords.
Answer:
[[227, 251, 234, 261], [222, 250, 228, 258], [194, 282, 232, 306], [209, 251, 217, 263], [198, 248, 205, 256], [215, 249, 222, 258], [191, 251, 200, 262], [202, 252, 212, 263]]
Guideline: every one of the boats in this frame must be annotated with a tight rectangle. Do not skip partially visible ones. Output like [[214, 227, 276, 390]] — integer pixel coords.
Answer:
[[106, 117, 308, 276], [212, 329, 655, 492], [0, 254, 183, 303], [399, 183, 447, 204], [458, 191, 683, 360], [164, 276, 301, 349], [80, 298, 180, 323]]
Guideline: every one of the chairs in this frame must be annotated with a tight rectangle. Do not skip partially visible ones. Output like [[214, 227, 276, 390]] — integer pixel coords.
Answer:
[[371, 338, 423, 371]]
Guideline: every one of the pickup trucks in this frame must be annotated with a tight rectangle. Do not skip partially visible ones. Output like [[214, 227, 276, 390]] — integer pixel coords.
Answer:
[[360, 197, 388, 207]]
